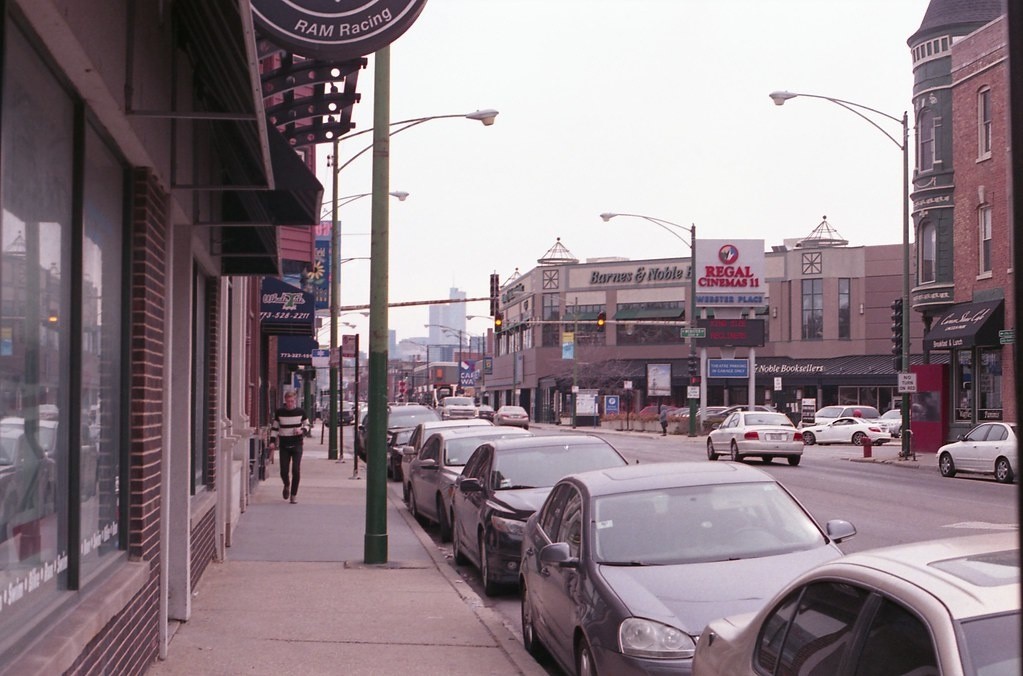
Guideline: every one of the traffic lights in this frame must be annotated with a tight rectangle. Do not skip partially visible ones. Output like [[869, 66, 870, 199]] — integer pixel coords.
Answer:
[[597, 311, 605, 326], [688, 354, 697, 375], [890, 298, 901, 372], [495, 315, 502, 332]]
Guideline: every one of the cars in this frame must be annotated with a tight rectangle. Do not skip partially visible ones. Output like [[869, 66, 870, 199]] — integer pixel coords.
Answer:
[[638, 404, 777, 433], [690, 531, 1023, 676], [707, 410, 805, 467], [448, 434, 635, 598], [406, 425, 536, 541], [800, 416, 891, 447], [935, 421, 1020, 484], [520, 459, 859, 676], [880, 409, 902, 438], [320, 396, 528, 504]]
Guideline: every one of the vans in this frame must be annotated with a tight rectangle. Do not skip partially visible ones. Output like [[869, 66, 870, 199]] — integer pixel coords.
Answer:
[[796, 405, 880, 436]]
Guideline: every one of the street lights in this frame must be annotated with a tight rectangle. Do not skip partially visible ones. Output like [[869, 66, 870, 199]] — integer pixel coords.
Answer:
[[328, 110, 499, 454], [513, 290, 578, 430], [599, 213, 700, 437], [408, 340, 430, 405], [767, 90, 912, 460], [465, 313, 516, 407]]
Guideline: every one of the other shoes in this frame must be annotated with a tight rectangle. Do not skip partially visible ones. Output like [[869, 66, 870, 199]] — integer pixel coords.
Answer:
[[660, 433, 667, 436], [290, 495, 297, 504], [283, 482, 291, 500]]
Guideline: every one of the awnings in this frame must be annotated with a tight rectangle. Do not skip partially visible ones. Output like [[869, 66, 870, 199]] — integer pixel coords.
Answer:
[[261, 274, 315, 336], [922, 299, 1004, 349], [278, 336, 319, 366]]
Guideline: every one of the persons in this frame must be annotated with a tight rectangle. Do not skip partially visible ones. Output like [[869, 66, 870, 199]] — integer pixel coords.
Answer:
[[659, 410, 667, 436], [270, 392, 310, 502]]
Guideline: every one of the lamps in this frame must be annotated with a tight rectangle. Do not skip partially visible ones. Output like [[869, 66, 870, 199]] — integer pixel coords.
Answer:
[[860, 303, 864, 314], [773, 306, 777, 318]]
